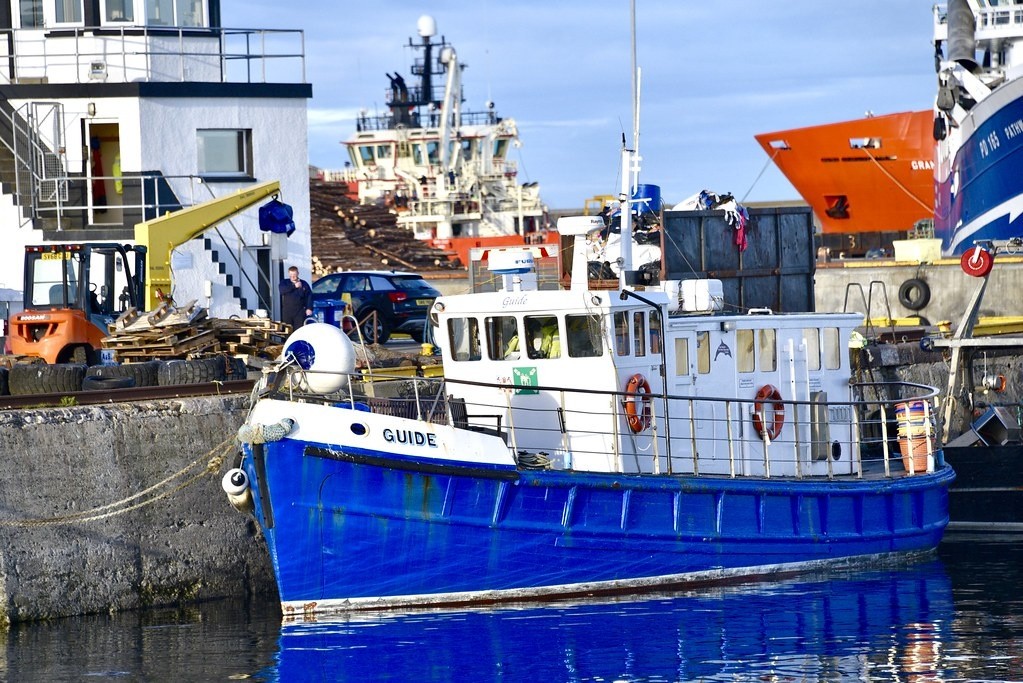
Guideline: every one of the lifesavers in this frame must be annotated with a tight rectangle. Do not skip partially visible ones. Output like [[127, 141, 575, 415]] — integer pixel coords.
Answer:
[[753, 384, 785, 441], [932, 117, 946, 140], [626, 372, 652, 433], [368, 162, 378, 172], [906, 315, 931, 326], [899, 279, 930, 310]]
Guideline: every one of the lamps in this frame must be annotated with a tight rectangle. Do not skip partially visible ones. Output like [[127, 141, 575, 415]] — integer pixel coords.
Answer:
[[721, 321, 737, 332], [89, 61, 107, 77]]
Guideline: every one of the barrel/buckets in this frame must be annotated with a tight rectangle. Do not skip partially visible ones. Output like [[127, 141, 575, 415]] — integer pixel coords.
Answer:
[[895, 400, 937, 472], [629, 184, 661, 215], [100, 349, 119, 366], [332, 402, 370, 412], [892, 239, 943, 262]]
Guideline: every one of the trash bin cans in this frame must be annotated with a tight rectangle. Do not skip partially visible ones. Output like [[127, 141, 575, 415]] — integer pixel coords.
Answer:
[[306, 299, 347, 330], [896, 400, 936, 473]]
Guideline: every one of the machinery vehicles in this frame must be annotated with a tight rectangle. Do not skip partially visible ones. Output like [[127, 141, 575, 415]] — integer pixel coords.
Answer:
[[5, 178, 282, 367]]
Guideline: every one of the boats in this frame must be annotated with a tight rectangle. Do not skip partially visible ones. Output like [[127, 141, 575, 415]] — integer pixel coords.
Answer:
[[222, 0, 958, 617], [316, 0, 1023, 534]]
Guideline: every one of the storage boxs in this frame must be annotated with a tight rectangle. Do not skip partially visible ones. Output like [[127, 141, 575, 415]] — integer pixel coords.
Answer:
[[659, 278, 724, 311]]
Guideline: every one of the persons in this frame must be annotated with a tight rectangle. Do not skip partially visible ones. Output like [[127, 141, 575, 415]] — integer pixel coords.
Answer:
[[279, 266, 313, 331]]
